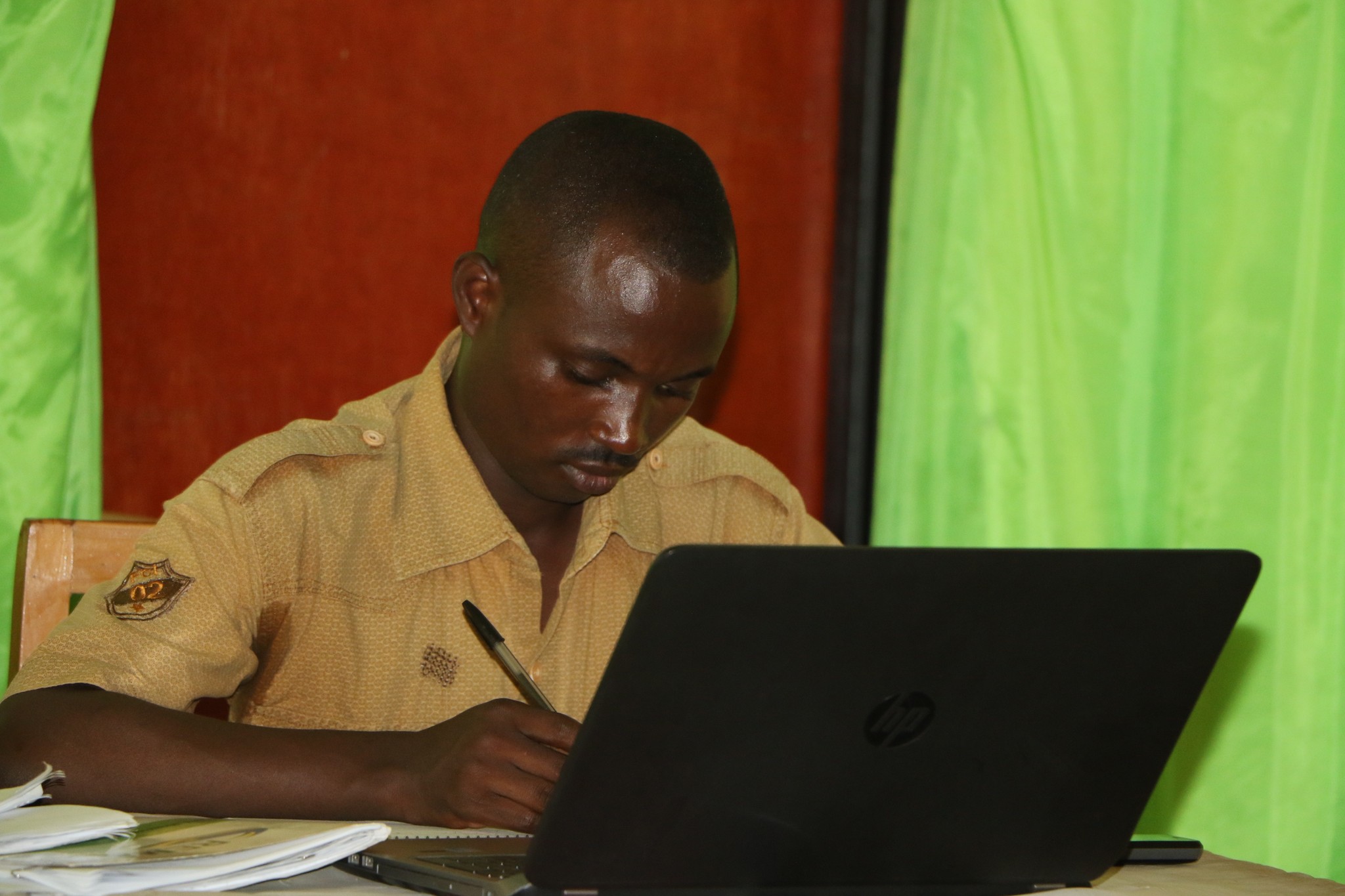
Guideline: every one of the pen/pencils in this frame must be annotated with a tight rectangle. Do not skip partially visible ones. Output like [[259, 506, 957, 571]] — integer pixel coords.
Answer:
[[462, 599, 557, 715]]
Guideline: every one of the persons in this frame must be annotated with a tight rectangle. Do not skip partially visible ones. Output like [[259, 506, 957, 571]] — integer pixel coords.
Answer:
[[1, 106, 851, 838]]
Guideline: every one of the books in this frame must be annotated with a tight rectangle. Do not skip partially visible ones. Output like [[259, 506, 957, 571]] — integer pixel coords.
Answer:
[[0, 760, 140, 855], [0, 815, 394, 895]]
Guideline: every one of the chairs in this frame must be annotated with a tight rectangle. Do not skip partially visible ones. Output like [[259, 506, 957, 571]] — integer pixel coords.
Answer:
[[5, 517, 159, 691]]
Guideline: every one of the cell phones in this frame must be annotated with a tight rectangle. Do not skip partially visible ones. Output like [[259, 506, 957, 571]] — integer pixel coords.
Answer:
[[1123, 833, 1205, 864]]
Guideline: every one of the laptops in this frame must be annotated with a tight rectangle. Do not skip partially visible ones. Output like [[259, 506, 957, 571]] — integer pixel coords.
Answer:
[[334, 542, 1263, 896]]
